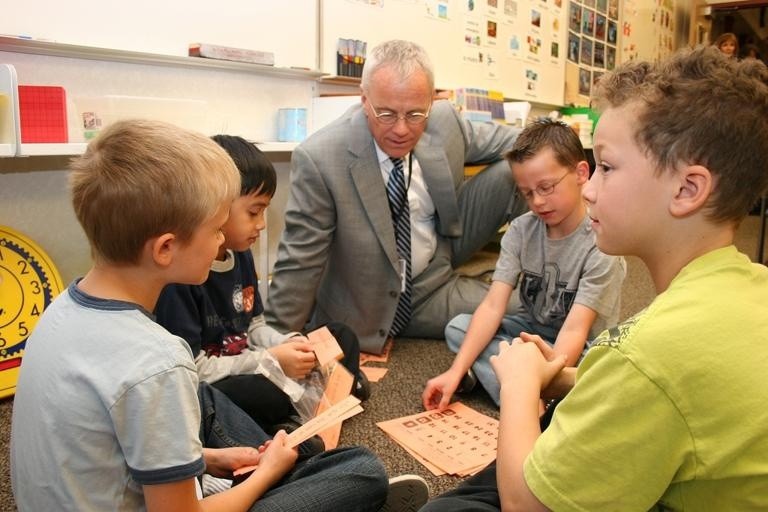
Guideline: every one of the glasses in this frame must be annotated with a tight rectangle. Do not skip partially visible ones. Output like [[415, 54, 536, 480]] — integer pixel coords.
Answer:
[[363, 96, 433, 121], [516, 172, 573, 201]]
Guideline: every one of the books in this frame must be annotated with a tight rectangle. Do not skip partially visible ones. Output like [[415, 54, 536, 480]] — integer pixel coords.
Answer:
[[275, 349, 356, 454]]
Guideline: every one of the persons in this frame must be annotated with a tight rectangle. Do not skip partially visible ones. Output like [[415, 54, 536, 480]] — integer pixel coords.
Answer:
[[261, 39, 534, 357], [9, 116, 431, 510], [149, 133, 374, 463], [420, 116, 632, 417], [421, 40, 768, 512], [713, 32, 741, 61]]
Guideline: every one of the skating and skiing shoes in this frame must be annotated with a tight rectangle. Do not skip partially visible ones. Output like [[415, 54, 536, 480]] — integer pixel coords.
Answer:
[[381, 474, 429, 512]]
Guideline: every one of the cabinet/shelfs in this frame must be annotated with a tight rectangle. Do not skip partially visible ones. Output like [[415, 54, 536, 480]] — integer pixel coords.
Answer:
[[0, 33, 568, 158]]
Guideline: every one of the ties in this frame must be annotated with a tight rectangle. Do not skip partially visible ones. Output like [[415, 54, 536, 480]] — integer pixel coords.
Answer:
[[386, 156, 412, 338]]
